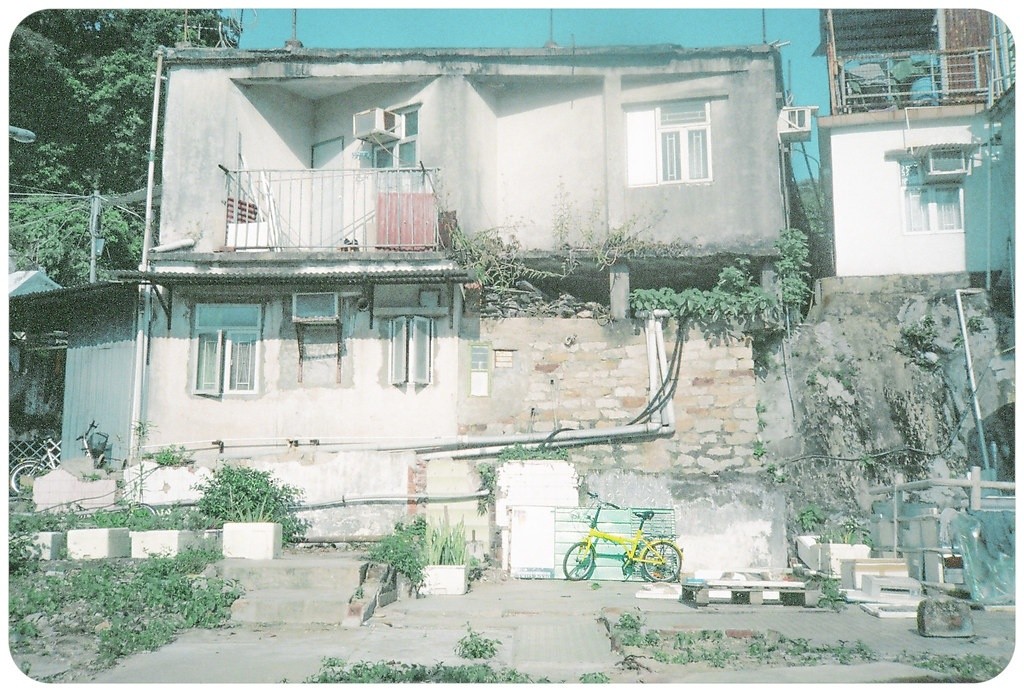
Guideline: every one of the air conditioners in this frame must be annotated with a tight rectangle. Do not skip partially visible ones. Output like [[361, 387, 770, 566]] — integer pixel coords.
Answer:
[[778, 109, 811, 142], [923, 149, 966, 183], [292, 291, 342, 324], [353, 107, 402, 145]]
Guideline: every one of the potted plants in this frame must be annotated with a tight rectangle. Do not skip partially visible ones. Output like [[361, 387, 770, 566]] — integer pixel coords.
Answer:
[[812, 517, 873, 574], [24, 502, 222, 563], [394, 521, 468, 595], [196, 462, 308, 562]]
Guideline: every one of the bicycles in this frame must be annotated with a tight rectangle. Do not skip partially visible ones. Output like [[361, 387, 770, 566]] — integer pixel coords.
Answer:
[[562, 493, 683, 584], [10, 419, 109, 495]]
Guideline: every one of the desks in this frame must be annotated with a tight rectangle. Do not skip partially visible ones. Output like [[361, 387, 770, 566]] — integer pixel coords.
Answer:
[[839, 557, 909, 590]]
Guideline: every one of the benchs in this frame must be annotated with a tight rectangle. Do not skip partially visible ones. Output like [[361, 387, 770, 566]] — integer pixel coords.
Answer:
[[731, 589, 764, 605], [862, 574, 922, 602], [682, 587, 710, 605], [780, 590, 819, 607]]
